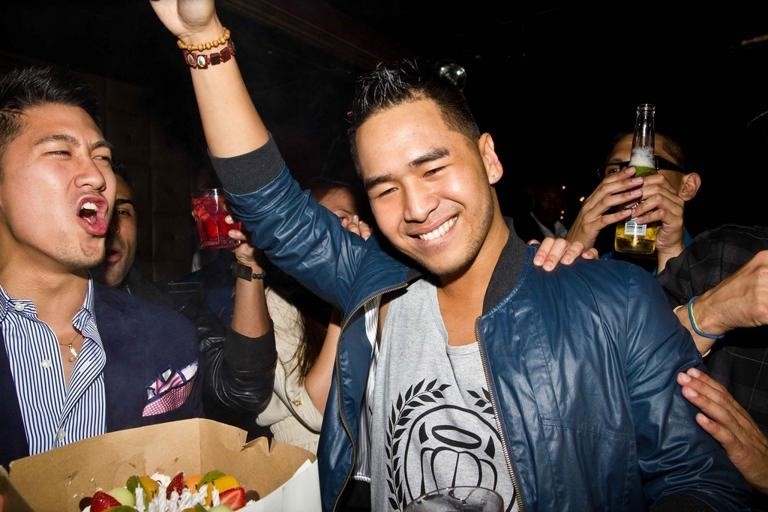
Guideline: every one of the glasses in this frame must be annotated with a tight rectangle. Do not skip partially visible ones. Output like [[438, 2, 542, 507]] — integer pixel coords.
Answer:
[[599, 155, 689, 178]]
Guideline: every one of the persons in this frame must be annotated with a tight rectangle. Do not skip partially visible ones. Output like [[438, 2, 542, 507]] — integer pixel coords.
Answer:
[[0, 0, 768, 512]]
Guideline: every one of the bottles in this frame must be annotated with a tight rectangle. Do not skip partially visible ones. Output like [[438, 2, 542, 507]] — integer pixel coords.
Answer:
[[614, 104, 660, 255]]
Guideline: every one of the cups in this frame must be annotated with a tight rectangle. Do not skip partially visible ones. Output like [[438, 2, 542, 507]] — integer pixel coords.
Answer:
[[190, 187, 242, 250]]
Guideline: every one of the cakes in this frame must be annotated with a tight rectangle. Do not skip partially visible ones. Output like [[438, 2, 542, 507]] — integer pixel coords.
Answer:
[[79, 470, 260, 512]]
